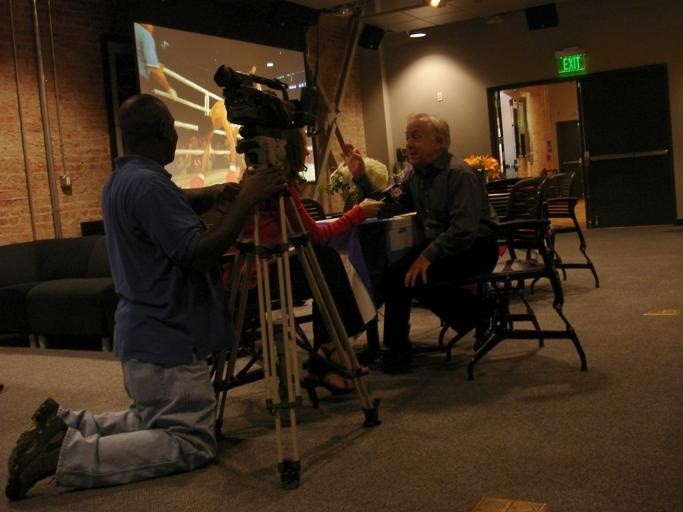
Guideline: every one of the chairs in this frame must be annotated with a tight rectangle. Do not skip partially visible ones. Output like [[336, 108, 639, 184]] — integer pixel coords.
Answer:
[[5, 417, 67, 501], [8, 397, 59, 477]]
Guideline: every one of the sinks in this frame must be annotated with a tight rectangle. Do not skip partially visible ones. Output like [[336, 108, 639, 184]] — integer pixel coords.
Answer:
[[0, 233, 116, 355]]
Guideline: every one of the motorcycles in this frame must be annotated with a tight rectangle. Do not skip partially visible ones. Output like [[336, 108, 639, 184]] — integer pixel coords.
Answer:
[[210, 137, 383, 490]]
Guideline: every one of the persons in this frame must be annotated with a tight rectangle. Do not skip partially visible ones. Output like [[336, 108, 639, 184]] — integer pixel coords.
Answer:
[[218, 124, 385, 409], [339, 113, 509, 375], [2, 92, 289, 506], [133, 23, 247, 189]]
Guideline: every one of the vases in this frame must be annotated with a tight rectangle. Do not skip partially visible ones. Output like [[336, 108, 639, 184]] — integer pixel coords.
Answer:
[[327, 156, 390, 196], [464, 150, 502, 183]]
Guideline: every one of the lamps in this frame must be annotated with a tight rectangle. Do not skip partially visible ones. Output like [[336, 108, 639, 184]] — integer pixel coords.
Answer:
[[179, 182, 322, 409], [439, 172, 602, 379]]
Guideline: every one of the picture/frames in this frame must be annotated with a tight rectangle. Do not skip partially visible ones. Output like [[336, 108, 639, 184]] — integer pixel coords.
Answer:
[[314, 210, 424, 360]]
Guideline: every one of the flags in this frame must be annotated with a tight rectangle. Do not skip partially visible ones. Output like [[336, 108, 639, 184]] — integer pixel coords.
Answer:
[[480, 174, 489, 184]]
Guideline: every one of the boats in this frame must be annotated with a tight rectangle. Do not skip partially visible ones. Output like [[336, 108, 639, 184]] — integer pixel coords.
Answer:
[[524, 3, 559, 31], [356, 23, 384, 50]]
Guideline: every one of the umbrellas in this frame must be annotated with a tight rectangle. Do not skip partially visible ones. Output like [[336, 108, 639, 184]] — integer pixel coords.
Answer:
[[473, 298, 498, 351]]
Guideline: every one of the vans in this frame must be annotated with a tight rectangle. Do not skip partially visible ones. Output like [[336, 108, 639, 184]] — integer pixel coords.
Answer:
[[301, 363, 355, 404], [314, 345, 368, 377]]
